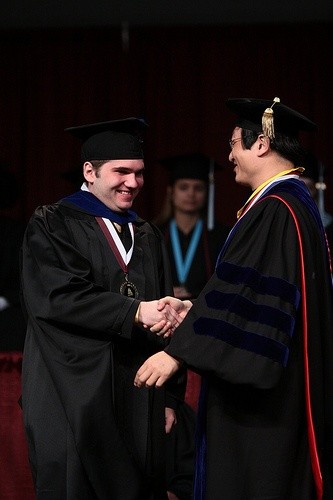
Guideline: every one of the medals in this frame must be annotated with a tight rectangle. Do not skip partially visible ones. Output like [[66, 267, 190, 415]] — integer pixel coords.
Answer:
[[96, 272, 139, 299]]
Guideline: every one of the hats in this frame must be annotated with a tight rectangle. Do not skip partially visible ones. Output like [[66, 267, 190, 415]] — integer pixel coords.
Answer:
[[225, 97, 319, 141], [64, 117, 145, 169], [295, 156, 328, 229], [168, 150, 225, 231]]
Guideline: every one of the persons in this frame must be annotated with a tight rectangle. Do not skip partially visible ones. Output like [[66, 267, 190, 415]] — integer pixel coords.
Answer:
[[21, 119, 184, 500], [153, 152, 227, 500], [133, 98, 333, 500]]
